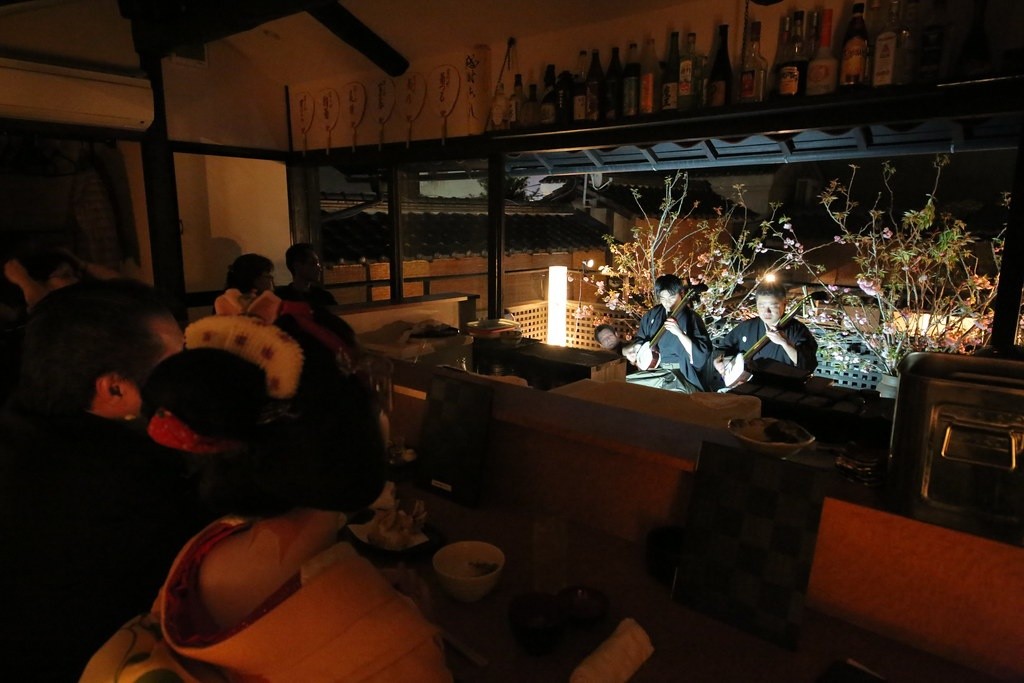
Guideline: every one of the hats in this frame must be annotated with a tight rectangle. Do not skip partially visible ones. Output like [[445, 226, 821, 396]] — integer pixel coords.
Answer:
[[141, 349, 268, 441]]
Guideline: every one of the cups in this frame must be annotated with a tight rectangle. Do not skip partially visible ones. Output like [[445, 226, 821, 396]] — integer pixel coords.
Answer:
[[507, 591, 564, 656]]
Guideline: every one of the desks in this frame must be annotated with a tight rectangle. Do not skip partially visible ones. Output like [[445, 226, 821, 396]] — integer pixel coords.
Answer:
[[470, 336, 542, 374], [339, 480, 1024, 683], [514, 345, 625, 392]]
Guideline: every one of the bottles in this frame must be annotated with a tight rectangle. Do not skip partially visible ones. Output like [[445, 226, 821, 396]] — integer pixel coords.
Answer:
[[489, 0, 1024, 131]]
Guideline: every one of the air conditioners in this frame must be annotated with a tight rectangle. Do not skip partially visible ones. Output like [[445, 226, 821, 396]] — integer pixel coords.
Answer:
[[0, 56, 156, 137]]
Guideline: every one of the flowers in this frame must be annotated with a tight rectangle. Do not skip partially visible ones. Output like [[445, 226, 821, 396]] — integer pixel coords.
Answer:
[[574, 151, 1012, 377]]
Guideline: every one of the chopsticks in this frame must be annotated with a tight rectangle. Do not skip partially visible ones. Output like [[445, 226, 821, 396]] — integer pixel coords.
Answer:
[[433, 623, 488, 668]]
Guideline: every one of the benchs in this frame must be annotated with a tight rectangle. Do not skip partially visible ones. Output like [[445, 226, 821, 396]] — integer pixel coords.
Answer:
[[362, 330, 474, 362]]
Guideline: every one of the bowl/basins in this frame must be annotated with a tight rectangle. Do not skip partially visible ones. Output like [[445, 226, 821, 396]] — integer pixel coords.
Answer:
[[547, 585, 610, 629], [431, 540, 506, 601], [728, 416, 817, 459], [499, 330, 523, 345], [465, 319, 521, 339]]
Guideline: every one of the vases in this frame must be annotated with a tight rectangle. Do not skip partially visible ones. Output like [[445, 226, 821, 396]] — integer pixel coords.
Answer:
[[875, 376, 899, 399]]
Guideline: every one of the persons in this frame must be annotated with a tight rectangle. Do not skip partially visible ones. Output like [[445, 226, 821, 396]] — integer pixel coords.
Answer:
[[711, 279, 818, 374], [594, 274, 714, 394], [0, 242, 456, 683]]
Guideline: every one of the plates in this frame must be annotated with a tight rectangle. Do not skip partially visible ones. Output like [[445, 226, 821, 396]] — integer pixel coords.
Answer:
[[348, 509, 429, 552]]
